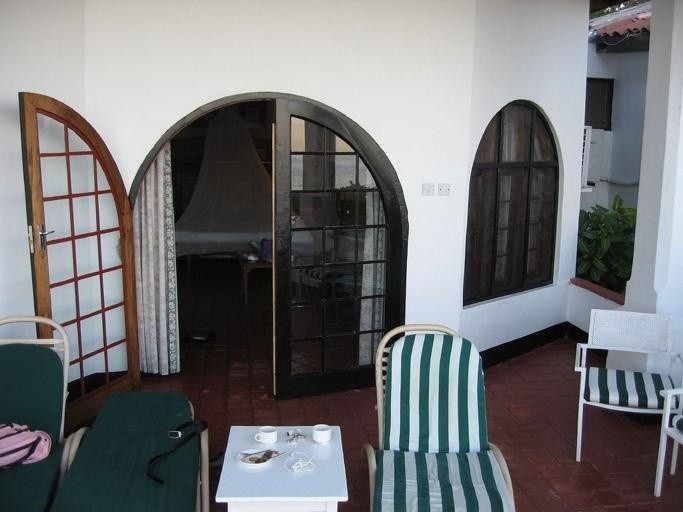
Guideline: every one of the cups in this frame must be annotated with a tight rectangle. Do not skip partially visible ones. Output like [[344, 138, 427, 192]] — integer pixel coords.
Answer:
[[312, 424, 331, 443], [252, 426, 278, 444]]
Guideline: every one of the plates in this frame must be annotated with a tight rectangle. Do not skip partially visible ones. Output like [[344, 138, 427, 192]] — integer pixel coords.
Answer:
[[237, 448, 271, 465]]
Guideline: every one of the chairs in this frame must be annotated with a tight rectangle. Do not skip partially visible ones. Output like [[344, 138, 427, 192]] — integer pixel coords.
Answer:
[[0, 318, 68, 512], [574, 310, 683, 476], [364, 325, 517, 512], [51, 389, 210, 512], [654, 381, 683, 497]]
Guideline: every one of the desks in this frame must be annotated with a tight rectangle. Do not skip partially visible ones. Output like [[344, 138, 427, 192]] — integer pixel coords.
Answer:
[[240, 255, 302, 305]]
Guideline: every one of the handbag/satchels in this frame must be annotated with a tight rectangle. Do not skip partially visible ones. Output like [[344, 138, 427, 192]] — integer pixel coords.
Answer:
[[0, 423, 41, 470]]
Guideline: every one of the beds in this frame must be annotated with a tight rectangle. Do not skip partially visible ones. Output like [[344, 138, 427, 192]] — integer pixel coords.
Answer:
[[175, 227, 313, 273]]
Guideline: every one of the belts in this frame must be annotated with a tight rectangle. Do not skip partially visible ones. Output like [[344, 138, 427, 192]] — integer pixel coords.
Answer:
[[146, 420, 207, 484]]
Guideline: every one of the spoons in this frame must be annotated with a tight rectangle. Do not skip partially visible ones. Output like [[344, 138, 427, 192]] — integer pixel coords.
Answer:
[[239, 449, 270, 456]]
